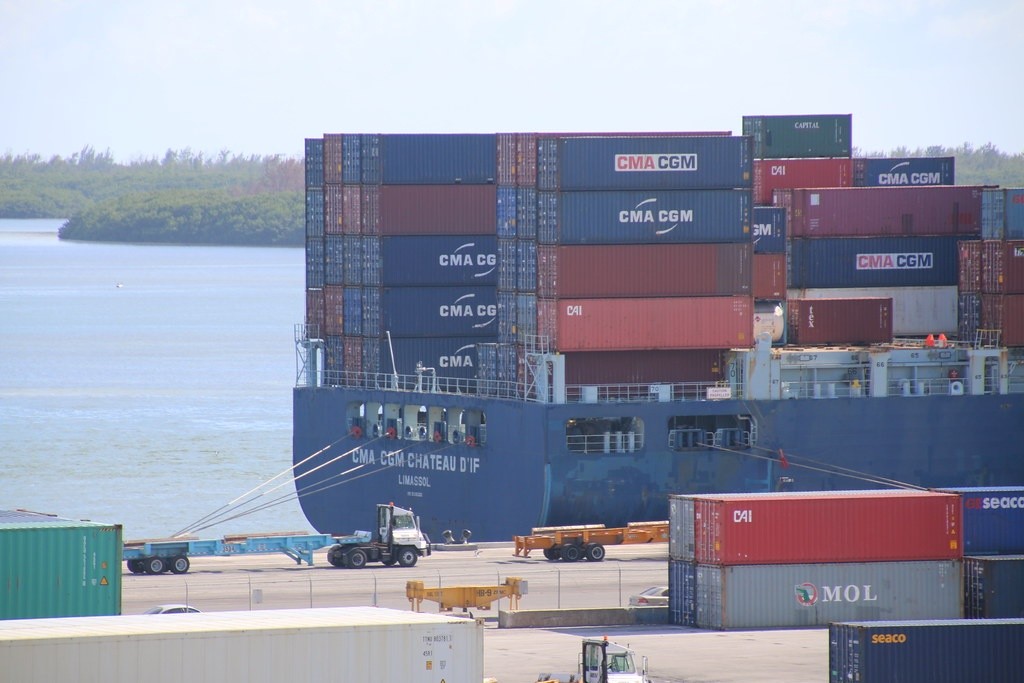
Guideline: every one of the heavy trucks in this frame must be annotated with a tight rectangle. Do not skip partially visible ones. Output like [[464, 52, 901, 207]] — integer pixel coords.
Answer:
[[122, 500, 433, 576]]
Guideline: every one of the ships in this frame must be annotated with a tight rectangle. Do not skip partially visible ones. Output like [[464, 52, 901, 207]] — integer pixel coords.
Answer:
[[289, 109, 1023, 546]]
